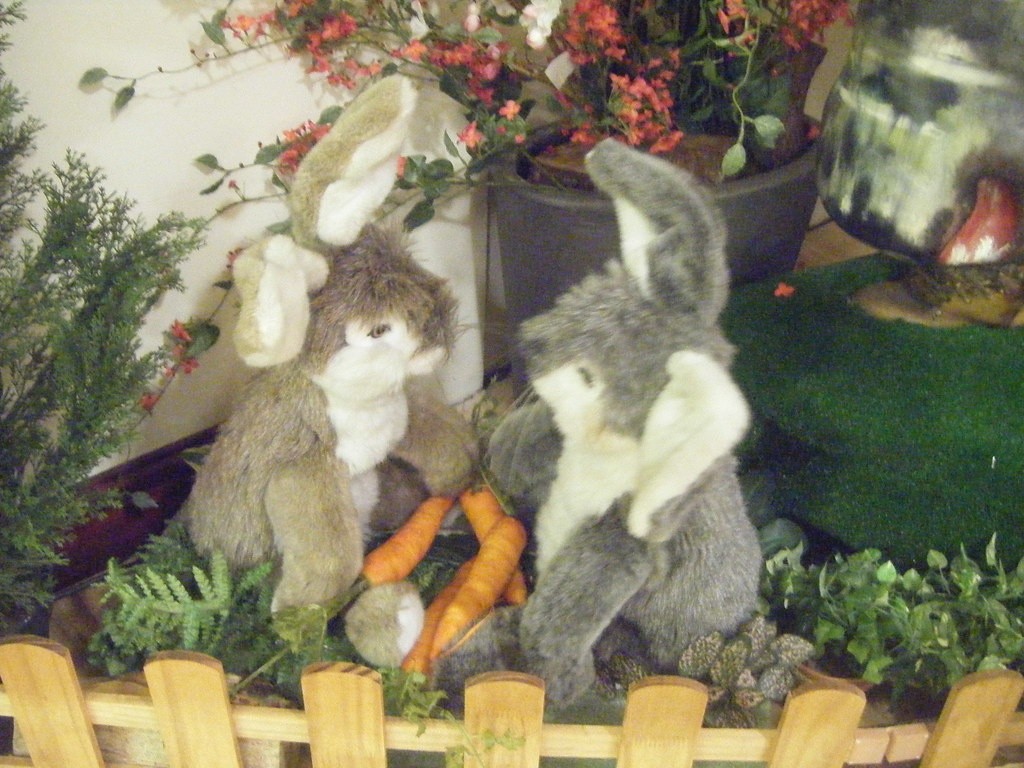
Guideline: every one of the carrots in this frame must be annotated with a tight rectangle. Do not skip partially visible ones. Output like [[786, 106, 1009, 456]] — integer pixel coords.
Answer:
[[357, 481, 527, 679]]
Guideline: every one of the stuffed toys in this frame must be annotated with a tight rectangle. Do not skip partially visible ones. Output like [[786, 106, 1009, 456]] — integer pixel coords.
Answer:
[[192, 74, 482, 650], [488, 134, 761, 710]]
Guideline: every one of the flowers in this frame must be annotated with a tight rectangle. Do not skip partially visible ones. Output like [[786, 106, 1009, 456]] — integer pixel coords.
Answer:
[[80, 0, 855, 434]]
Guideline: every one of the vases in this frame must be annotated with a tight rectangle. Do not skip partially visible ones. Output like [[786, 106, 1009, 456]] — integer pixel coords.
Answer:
[[488, 114, 821, 408]]
